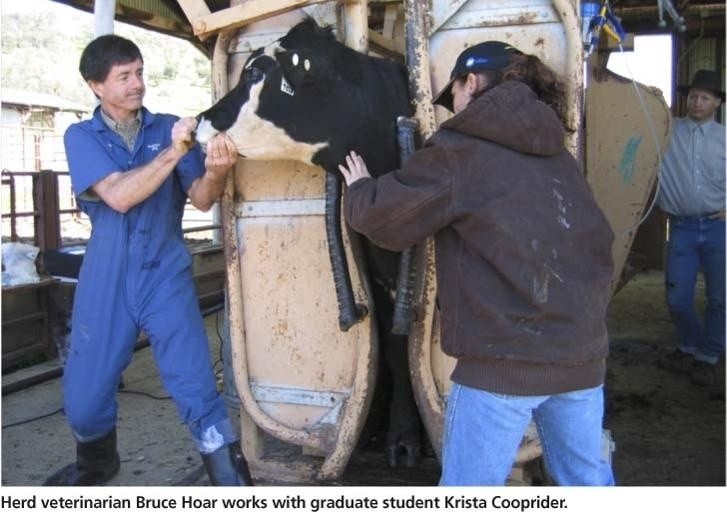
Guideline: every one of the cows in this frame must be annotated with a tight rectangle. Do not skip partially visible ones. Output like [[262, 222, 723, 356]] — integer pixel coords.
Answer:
[[189, 6, 421, 470]]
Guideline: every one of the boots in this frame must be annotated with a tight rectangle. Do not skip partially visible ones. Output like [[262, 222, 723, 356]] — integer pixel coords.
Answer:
[[202, 441, 253, 485], [42, 425, 119, 485]]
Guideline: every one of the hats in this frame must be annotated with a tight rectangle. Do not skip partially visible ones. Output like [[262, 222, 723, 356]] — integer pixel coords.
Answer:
[[676, 71, 725, 102], [430, 41, 522, 114]]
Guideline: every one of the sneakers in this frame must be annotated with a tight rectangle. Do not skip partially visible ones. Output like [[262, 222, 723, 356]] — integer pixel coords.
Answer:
[[658, 348, 712, 385]]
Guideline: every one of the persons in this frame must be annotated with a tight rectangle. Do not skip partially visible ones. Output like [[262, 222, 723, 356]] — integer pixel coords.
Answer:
[[648, 69, 725, 371], [332, 41, 615, 486], [39, 34, 256, 486]]
